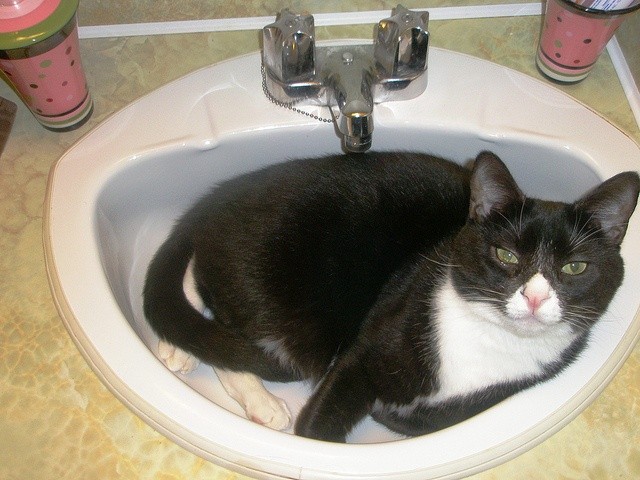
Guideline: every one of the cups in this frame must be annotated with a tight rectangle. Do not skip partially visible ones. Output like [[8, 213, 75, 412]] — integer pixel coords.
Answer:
[[0, 0, 95, 131], [536, 0, 634, 84]]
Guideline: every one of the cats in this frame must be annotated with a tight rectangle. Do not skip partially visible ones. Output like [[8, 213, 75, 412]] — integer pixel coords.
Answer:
[[141, 149, 640, 443]]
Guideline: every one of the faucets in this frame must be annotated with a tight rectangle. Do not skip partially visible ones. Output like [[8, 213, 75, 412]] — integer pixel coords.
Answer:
[[324, 71, 378, 152]]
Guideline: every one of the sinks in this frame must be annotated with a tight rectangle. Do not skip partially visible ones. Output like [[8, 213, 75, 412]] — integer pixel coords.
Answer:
[[94, 124, 604, 445]]
